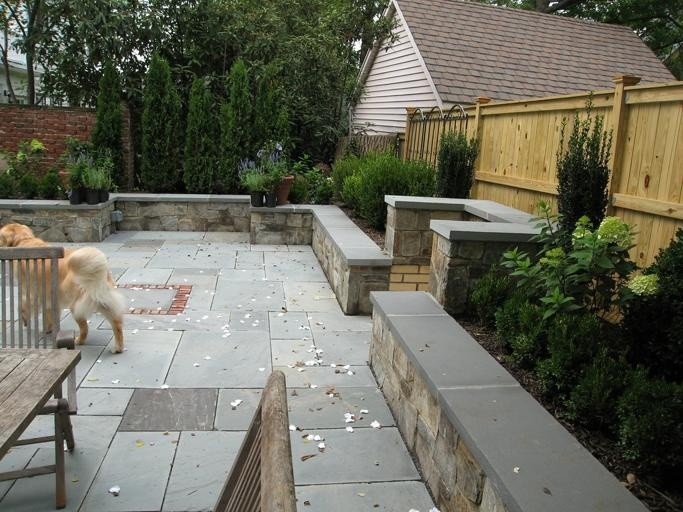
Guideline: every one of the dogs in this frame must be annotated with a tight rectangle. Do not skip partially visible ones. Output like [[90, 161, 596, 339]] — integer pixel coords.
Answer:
[[0, 223, 126, 355]]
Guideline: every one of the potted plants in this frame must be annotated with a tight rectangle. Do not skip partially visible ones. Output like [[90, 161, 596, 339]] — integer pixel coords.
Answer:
[[65, 143, 114, 205], [239, 158, 295, 207]]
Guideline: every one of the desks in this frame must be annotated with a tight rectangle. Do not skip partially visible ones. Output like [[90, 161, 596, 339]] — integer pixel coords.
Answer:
[[2, 345, 80, 511]]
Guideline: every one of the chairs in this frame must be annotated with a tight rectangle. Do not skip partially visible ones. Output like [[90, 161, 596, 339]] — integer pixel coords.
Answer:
[[2, 248, 86, 510], [200, 370, 300, 512]]
